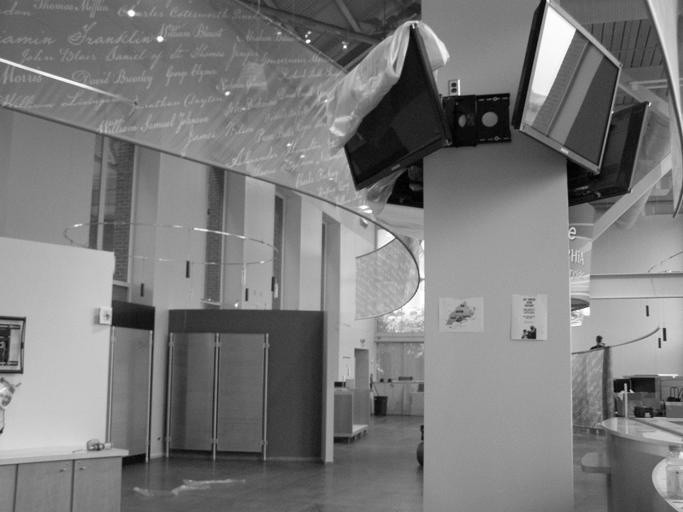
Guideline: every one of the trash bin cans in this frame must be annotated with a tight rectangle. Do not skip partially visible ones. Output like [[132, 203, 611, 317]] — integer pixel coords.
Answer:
[[374, 396, 388, 416]]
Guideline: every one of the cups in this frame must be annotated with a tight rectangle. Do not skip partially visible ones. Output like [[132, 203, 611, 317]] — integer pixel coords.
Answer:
[[644, 413, 649, 417]]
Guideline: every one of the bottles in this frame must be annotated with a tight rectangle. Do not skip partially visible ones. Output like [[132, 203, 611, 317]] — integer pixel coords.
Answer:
[[664, 445, 683, 499], [89, 442, 112, 451]]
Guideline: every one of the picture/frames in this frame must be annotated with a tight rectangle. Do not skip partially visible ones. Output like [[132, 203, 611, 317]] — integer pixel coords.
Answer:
[[0, 314, 29, 375]]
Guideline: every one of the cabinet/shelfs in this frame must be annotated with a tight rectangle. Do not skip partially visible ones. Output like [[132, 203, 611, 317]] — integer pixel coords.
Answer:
[[0, 441, 131, 512]]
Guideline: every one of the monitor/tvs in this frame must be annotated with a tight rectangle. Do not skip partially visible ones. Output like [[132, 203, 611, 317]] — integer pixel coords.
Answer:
[[367, 158, 423, 208], [343, 23, 452, 191], [511, 0, 624, 176], [568, 101, 650, 206]]
